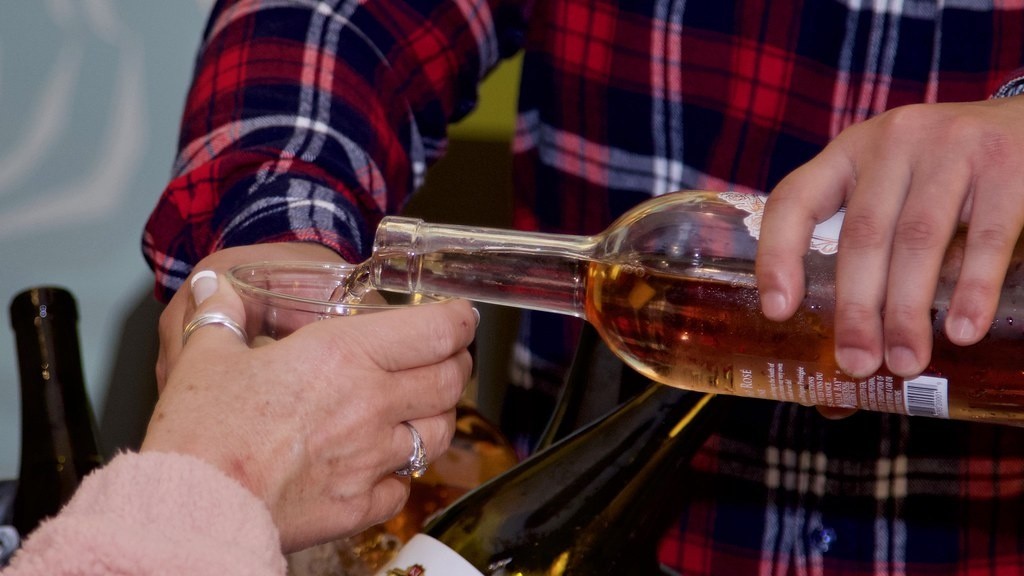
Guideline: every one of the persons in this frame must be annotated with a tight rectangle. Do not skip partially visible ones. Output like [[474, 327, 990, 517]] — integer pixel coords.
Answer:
[[139, 0, 1024, 576], [1, 271, 479, 575]]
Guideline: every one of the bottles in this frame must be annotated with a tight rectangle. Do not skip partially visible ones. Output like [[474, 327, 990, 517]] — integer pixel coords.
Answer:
[[371, 190, 1024, 428], [374, 381, 726, 576], [0, 286, 102, 572]]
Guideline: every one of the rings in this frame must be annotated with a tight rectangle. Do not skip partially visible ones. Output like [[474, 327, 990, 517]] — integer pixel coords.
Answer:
[[394, 421, 428, 477], [184, 315, 248, 345]]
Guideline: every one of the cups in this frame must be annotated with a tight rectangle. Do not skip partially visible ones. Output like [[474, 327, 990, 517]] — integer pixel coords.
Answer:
[[224, 260, 454, 576]]
